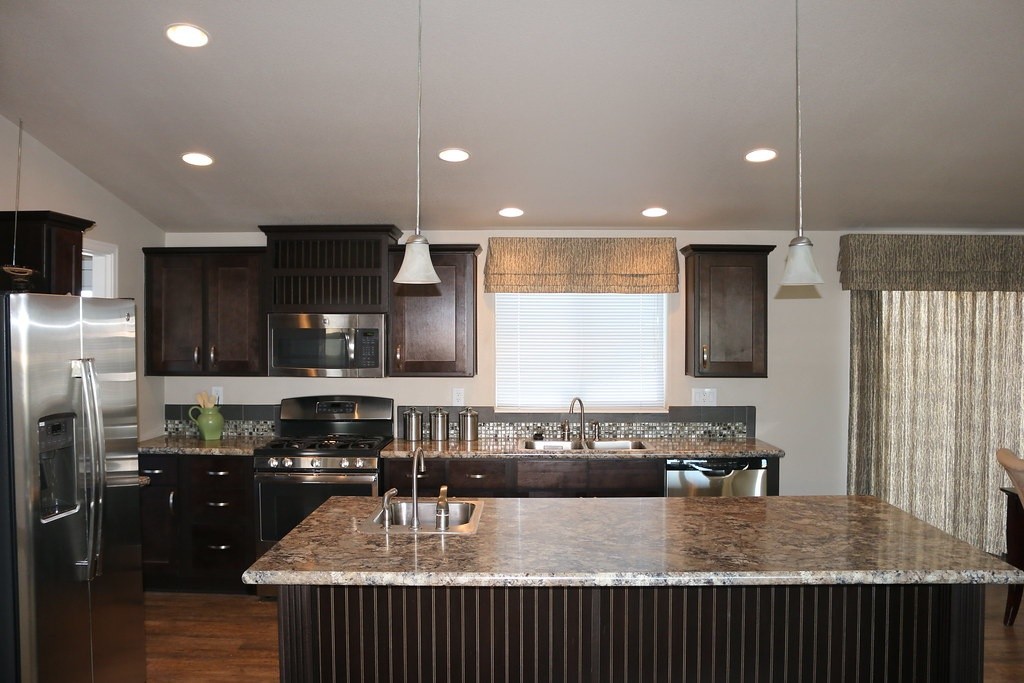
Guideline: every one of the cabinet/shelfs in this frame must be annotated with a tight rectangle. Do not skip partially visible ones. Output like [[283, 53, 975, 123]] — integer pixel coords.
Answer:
[[680, 244, 777, 378], [517, 460, 669, 498], [138, 454, 257, 597], [383, 458, 518, 498], [0, 209, 96, 296], [142, 246, 270, 377], [388, 243, 484, 379]]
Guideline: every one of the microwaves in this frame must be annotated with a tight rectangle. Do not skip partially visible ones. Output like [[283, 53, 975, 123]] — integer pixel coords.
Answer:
[[264, 313, 388, 379]]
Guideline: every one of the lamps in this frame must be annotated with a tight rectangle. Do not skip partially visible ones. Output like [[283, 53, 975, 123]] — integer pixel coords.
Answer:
[[392, 0, 442, 286], [779, 0, 825, 289]]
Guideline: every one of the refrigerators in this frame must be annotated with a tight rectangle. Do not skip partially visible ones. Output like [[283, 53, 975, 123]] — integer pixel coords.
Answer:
[[0, 291, 148, 683]]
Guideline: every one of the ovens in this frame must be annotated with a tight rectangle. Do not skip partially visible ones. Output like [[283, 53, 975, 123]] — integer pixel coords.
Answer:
[[252, 472, 383, 554]]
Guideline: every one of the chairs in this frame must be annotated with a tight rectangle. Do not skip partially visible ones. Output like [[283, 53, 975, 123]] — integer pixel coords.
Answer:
[[996, 447, 1024, 626]]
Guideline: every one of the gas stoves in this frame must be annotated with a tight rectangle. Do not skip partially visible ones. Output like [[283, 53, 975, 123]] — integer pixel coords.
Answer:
[[252, 394, 394, 470]]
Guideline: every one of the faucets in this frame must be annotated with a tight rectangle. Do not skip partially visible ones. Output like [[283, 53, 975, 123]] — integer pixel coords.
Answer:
[[585, 439, 646, 449], [568, 397, 585, 441], [411, 445, 427, 532]]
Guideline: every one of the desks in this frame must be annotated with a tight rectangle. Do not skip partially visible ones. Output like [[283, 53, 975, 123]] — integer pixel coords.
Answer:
[[241, 494, 1024, 683]]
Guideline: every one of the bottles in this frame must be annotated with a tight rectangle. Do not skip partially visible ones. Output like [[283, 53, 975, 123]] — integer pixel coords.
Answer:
[[458, 407, 479, 441], [402, 407, 423, 441], [429, 407, 450, 442]]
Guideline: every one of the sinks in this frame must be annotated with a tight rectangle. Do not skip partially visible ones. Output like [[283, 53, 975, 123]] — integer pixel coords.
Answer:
[[523, 440, 585, 449], [372, 499, 476, 526]]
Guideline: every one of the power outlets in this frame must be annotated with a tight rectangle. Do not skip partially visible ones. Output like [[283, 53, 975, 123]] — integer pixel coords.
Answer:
[[212, 386, 223, 404], [692, 388, 718, 406]]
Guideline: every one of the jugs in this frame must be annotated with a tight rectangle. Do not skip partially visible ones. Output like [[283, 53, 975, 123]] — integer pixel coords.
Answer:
[[188, 406, 224, 441]]
